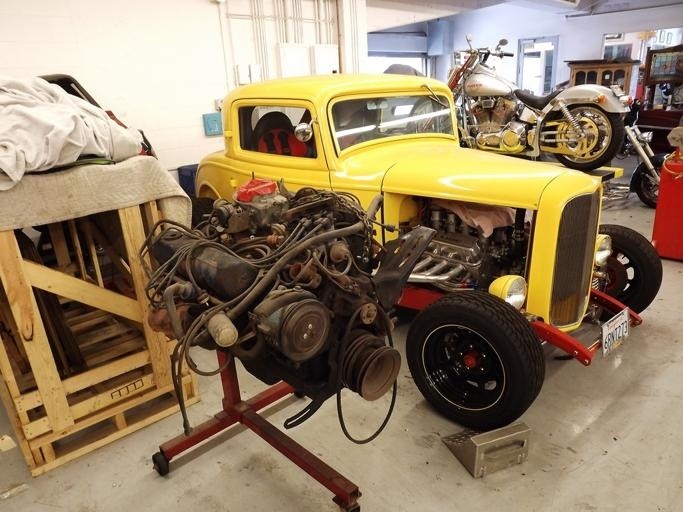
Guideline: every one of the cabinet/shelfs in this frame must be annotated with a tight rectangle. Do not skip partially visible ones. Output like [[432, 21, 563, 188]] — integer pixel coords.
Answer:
[[638, 44, 683, 154], [569, 64, 639, 99]]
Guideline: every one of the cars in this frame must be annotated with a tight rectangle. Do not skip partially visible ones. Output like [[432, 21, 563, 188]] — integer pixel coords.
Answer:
[[194, 72, 664, 434]]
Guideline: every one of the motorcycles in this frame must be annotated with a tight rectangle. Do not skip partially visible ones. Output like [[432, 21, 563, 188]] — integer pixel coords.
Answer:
[[405, 33, 634, 173], [624, 98, 670, 210]]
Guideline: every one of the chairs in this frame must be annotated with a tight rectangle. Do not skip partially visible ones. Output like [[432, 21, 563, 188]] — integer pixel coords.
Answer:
[[252, 111, 307, 156]]
